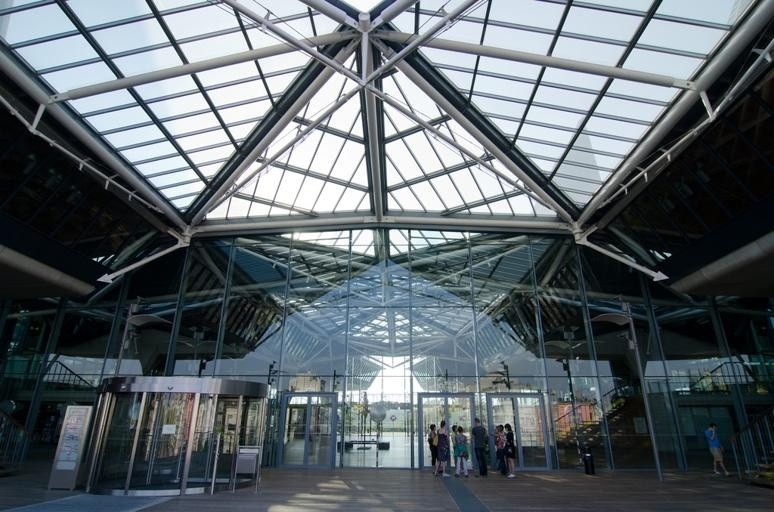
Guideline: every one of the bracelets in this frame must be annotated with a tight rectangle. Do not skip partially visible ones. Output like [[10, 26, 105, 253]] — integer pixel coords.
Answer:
[[712, 431, 715, 433]]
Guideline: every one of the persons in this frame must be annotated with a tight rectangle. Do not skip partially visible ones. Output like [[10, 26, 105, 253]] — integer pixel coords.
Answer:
[[428, 417, 517, 480], [704, 422, 733, 476]]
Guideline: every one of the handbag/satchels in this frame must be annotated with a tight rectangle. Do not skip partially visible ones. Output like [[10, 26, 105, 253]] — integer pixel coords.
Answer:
[[505, 443, 513, 455], [432, 429, 439, 446]]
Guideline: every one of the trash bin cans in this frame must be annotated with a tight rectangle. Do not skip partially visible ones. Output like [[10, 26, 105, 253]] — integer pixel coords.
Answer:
[[581, 442, 595, 475]]
